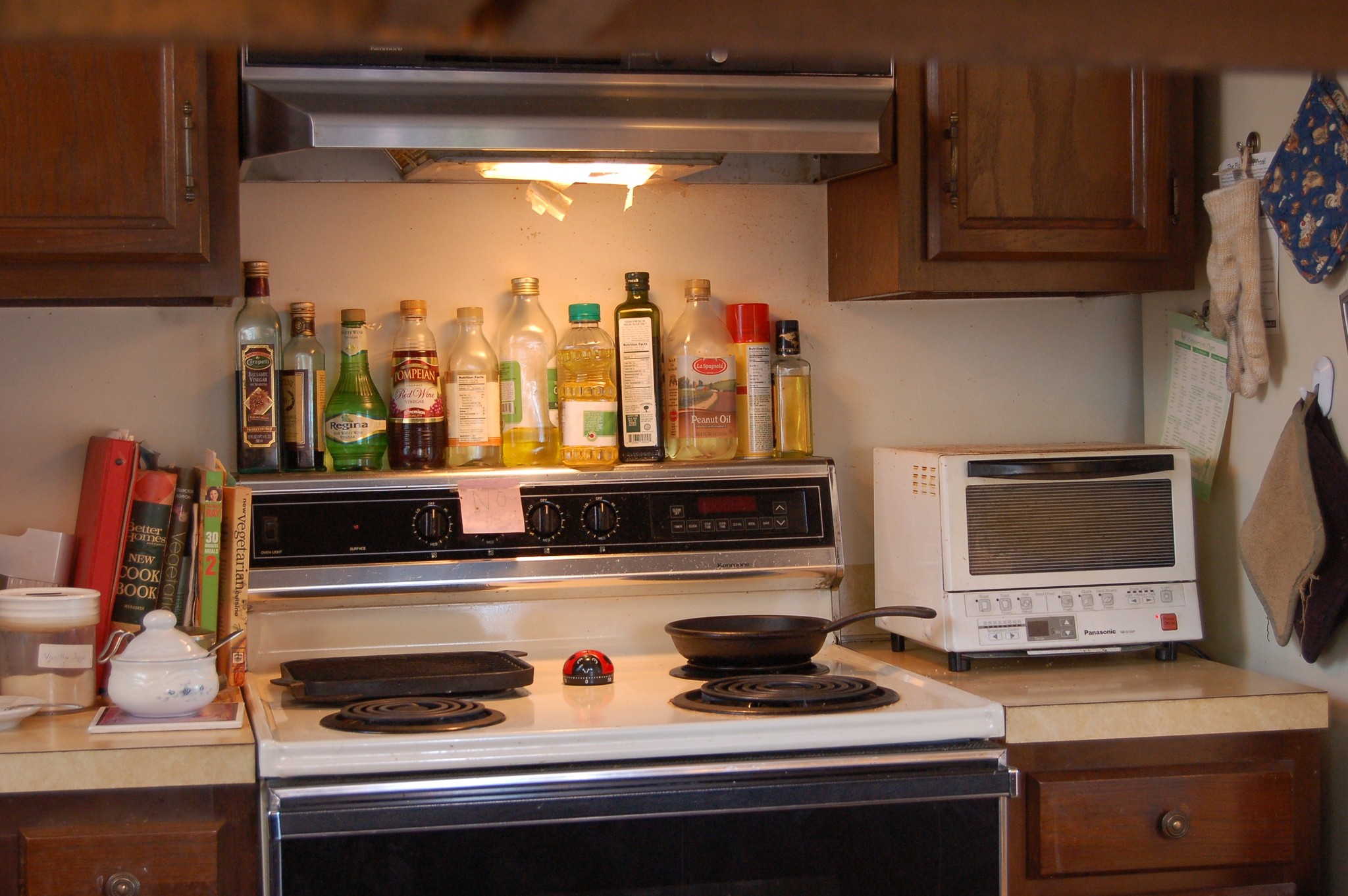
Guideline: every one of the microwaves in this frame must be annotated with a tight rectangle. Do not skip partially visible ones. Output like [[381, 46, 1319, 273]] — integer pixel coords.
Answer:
[[873, 445, 1201, 672]]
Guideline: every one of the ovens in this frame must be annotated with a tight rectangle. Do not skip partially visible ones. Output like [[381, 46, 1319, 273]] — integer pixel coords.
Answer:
[[248, 741, 1014, 895]]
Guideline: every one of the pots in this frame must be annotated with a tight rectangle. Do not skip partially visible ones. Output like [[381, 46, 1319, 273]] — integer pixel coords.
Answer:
[[663, 604, 938, 663]]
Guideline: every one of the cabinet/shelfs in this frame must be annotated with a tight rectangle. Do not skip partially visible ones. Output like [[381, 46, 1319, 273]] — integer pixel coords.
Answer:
[[1005, 730, 1330, 894], [0, 784, 262, 896], [828, 60, 1194, 302], [0, 37, 244, 309]]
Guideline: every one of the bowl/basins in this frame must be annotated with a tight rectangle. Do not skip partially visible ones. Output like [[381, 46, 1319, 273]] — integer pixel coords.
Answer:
[[105, 611, 221, 717]]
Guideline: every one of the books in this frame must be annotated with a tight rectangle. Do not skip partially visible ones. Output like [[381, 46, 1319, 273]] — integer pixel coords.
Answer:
[[68, 435, 252, 690]]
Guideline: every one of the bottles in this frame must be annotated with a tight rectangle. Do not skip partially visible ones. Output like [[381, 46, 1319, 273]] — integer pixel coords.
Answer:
[[1, 585, 101, 711], [233, 257, 815, 475]]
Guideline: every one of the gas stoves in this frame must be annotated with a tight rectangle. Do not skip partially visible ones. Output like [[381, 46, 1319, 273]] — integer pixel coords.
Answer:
[[242, 636, 1009, 767]]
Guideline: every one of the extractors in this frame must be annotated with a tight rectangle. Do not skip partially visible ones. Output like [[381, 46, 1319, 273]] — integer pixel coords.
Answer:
[[237, 40, 897, 192]]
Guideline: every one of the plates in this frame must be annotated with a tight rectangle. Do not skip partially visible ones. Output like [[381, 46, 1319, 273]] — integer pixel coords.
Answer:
[[266, 647, 535, 700], [0, 696, 44, 729]]
